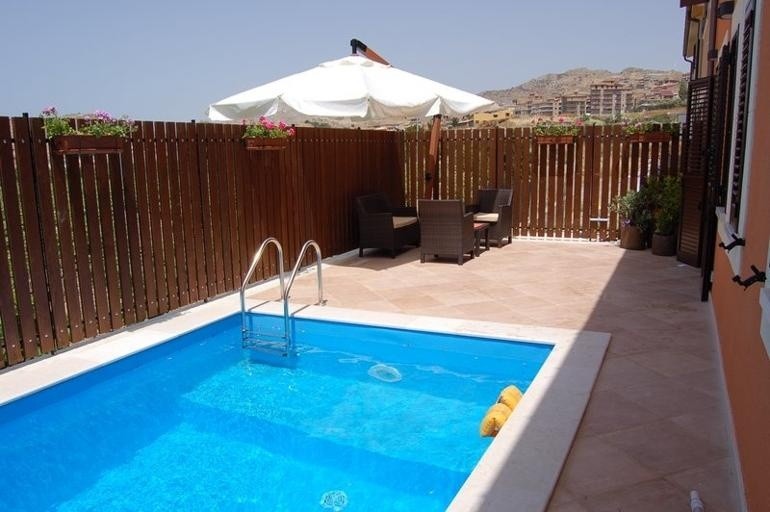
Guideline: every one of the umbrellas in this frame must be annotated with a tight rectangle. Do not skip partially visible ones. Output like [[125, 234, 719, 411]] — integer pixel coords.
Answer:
[[208, 43, 498, 129]]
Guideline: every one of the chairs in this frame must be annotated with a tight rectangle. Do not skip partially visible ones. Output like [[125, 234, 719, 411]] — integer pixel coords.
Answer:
[[465, 187, 514, 248], [354, 191, 420, 259], [418, 197, 476, 267]]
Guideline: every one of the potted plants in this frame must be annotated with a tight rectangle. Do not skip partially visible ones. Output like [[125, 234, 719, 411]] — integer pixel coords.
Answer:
[[639, 166, 685, 258], [607, 184, 651, 252]]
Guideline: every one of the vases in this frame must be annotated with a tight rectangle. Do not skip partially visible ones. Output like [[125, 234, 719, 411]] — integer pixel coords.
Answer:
[[53, 136, 126, 155], [244, 135, 287, 150], [536, 135, 575, 145]]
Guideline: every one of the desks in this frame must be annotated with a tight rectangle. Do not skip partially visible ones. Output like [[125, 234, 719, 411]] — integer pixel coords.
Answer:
[[473, 222, 491, 257]]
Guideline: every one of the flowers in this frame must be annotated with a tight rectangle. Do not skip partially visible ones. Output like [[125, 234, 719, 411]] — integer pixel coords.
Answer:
[[529, 115, 587, 139], [38, 104, 137, 139], [239, 115, 297, 140]]
